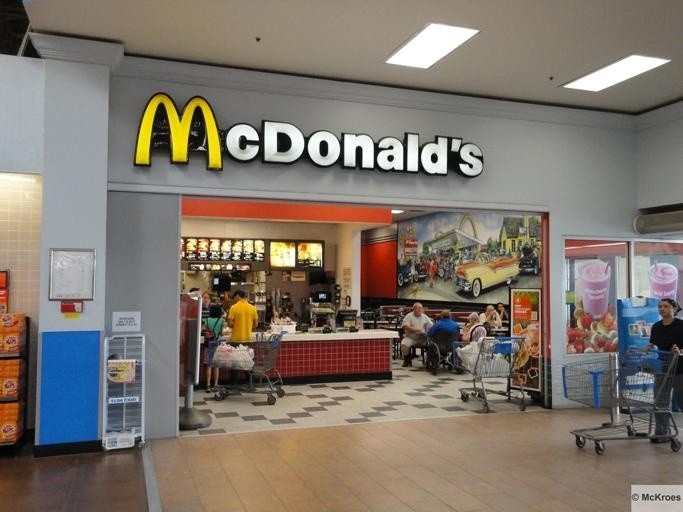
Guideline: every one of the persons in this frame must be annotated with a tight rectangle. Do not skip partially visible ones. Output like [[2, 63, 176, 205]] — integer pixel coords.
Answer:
[[410, 249, 500, 289], [400, 302, 435, 368], [495, 302, 509, 320], [227, 289, 259, 387], [642, 298, 683, 443], [204, 303, 225, 393], [479, 304, 503, 329], [462, 312, 487, 343], [201, 290, 228, 329], [427, 309, 461, 350]]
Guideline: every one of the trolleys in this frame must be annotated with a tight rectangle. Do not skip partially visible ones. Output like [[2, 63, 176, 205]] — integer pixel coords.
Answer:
[[451, 336, 527, 414], [208, 329, 288, 405], [561, 344, 682, 456]]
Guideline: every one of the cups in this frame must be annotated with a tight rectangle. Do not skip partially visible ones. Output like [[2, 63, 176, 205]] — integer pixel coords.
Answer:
[[578, 261, 611, 320], [648, 263, 678, 302]]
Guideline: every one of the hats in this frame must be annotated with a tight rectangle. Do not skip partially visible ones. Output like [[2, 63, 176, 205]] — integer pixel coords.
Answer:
[[231, 289, 245, 299]]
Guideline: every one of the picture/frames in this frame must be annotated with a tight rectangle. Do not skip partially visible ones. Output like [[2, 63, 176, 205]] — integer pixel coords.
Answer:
[[48, 248, 97, 302]]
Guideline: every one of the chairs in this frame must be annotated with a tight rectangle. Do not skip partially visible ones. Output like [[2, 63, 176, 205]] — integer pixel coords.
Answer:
[[337, 306, 511, 367]]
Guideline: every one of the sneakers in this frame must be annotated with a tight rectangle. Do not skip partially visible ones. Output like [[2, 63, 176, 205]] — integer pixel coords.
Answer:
[[403, 358, 411, 366], [204, 386, 211, 392]]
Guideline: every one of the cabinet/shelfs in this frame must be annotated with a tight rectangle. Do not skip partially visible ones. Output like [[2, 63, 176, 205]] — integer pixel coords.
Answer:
[[0, 318, 30, 445]]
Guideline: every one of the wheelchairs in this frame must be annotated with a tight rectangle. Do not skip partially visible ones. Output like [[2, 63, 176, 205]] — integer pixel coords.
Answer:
[[422, 326, 466, 375]]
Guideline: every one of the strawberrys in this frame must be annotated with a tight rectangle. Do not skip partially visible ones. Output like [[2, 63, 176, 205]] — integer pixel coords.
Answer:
[[566, 307, 618, 353]]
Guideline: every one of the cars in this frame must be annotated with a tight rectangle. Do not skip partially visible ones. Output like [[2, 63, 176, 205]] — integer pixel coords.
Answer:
[[453, 244, 541, 298], [398, 258, 446, 288]]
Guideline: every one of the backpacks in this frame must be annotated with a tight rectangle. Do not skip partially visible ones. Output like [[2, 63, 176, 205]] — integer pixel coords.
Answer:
[[204, 317, 222, 341]]
